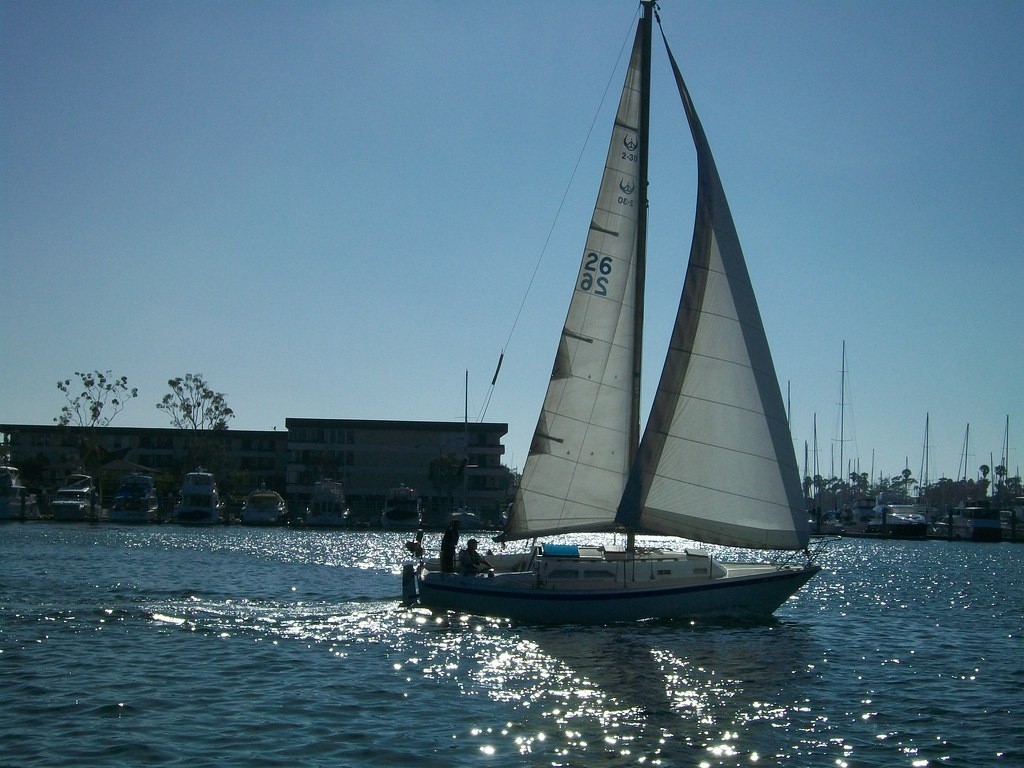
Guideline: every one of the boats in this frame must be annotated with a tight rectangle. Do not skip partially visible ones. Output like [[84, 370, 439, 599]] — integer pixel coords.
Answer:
[[47, 473, 96, 524], [172, 468, 219, 524], [447, 503, 480, 531], [308, 478, 348, 527], [865, 490, 928, 540], [933, 506, 1002, 542], [239, 488, 290, 527], [111, 472, 160, 523], [379, 482, 424, 528]]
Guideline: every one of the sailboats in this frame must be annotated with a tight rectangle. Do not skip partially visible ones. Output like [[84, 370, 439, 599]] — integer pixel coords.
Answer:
[[400, 1, 823, 630]]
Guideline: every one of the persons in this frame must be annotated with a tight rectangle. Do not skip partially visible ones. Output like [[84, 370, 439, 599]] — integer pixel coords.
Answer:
[[459, 539, 497, 576], [441, 520, 461, 572]]
